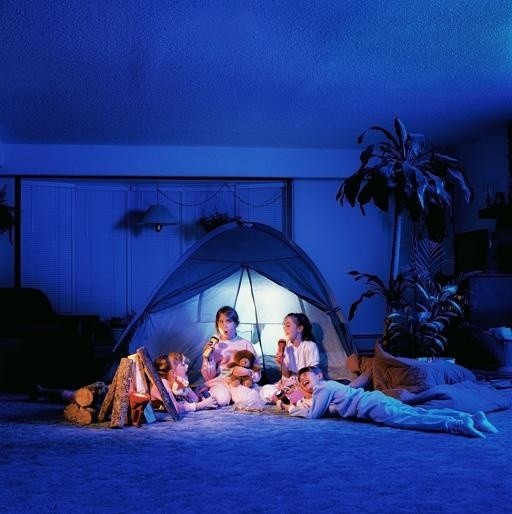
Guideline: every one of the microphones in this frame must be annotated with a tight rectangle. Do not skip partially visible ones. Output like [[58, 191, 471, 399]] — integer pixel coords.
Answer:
[[278, 339, 286, 353], [202, 334, 219, 358]]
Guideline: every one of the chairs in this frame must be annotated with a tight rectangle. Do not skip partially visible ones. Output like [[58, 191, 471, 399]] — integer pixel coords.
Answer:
[[1, 287, 114, 395]]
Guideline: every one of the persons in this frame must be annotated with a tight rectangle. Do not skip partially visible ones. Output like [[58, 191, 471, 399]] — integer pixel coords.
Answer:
[[29, 352, 218, 412], [200, 306, 320, 407], [282, 367, 498, 438]]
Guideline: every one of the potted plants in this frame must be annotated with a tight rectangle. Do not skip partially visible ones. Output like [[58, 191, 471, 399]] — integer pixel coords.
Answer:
[[385, 302, 459, 364], [105, 315, 125, 346]]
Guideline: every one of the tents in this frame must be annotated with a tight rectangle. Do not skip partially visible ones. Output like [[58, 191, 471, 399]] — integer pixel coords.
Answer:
[[99, 221, 360, 385]]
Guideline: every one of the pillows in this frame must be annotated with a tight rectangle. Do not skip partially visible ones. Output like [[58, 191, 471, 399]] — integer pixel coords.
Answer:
[[370, 338, 476, 402]]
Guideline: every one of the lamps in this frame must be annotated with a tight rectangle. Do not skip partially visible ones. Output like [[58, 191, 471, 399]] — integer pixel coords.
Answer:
[[136, 183, 181, 232]]
